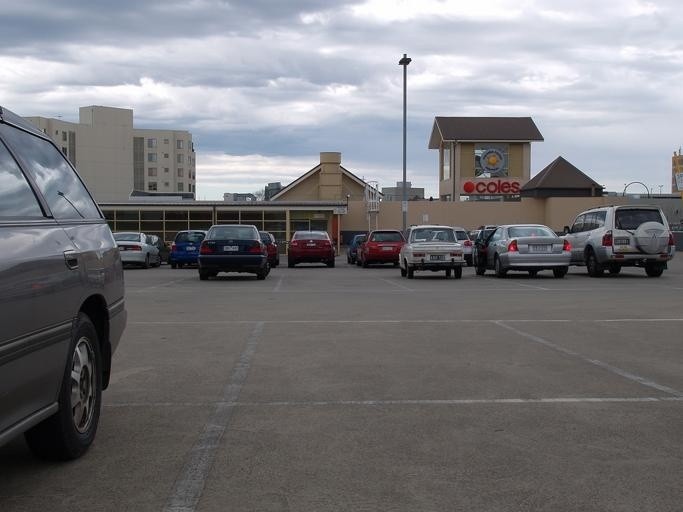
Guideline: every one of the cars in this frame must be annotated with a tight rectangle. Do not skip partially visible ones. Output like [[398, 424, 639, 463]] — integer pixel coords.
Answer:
[[397, 225, 467, 282], [473, 224, 573, 278], [191, 225, 272, 279]]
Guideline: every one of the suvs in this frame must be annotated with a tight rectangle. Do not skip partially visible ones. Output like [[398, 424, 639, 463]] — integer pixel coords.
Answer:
[[557, 203, 676, 278], [0, 107, 127, 461]]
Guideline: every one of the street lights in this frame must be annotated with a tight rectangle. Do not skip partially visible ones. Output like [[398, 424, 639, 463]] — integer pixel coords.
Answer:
[[658, 184, 663, 198], [398, 53, 410, 233]]
